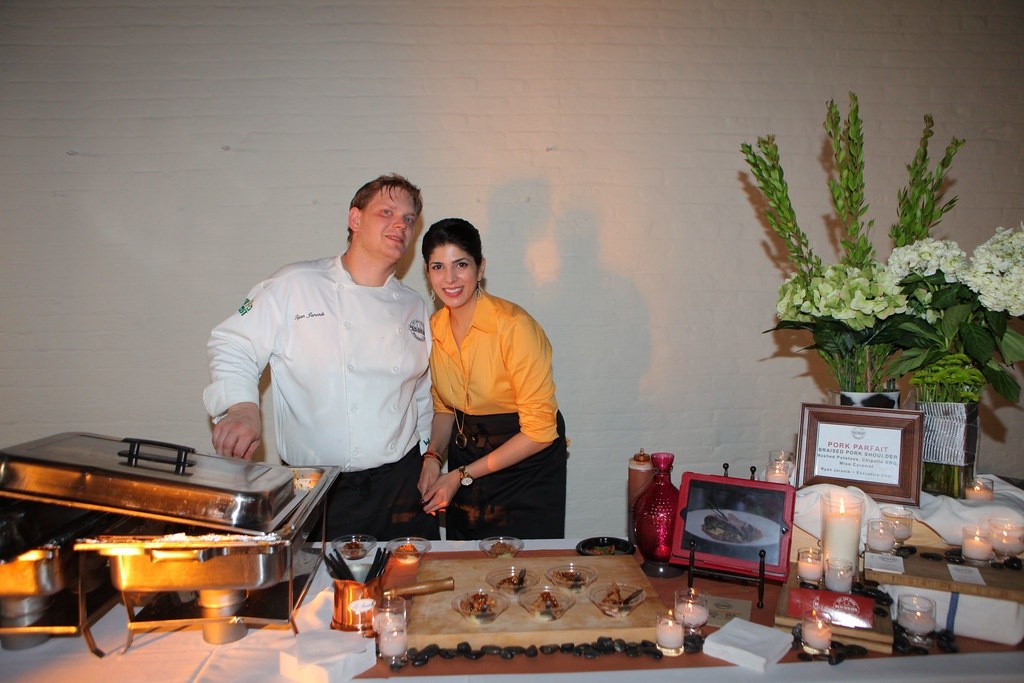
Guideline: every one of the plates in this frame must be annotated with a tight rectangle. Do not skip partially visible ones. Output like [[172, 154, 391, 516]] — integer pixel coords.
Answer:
[[685, 510, 781, 547]]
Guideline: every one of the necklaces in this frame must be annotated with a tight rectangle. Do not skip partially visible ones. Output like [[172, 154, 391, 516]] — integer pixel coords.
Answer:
[[448, 339, 477, 450]]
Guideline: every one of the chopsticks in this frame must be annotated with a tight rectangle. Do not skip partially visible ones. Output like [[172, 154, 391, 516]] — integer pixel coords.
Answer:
[[323, 547, 393, 583]]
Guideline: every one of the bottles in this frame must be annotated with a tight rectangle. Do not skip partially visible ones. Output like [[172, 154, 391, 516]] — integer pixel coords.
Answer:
[[628, 448, 655, 547]]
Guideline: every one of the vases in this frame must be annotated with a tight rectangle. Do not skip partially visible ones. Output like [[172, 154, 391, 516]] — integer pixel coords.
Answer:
[[916, 402, 982, 500], [839, 391, 901, 409]]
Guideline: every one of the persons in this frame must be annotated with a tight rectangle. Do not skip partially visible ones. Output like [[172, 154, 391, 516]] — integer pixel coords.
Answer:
[[418, 217, 568, 542], [202, 171, 442, 542]]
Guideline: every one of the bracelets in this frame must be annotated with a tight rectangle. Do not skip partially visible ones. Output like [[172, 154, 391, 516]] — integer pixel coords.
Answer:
[[424, 450, 443, 469]]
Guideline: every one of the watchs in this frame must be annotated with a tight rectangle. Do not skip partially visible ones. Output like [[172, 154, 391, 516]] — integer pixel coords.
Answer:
[[459, 465, 473, 486]]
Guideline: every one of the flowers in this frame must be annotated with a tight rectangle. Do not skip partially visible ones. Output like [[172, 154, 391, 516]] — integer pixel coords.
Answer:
[[739, 91, 1024, 403]]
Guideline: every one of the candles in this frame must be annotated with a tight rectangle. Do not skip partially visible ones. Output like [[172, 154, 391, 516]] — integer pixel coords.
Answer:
[[371, 595, 408, 665], [961, 518, 1024, 568], [657, 492, 937, 656], [767, 450, 794, 485], [964, 477, 994, 501]]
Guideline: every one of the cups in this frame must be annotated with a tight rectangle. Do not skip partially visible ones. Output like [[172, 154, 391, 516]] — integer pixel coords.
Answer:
[[330, 576, 383, 637], [373, 596, 406, 663]]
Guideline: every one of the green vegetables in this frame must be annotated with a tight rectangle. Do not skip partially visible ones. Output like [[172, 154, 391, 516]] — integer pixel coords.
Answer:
[[594, 547, 624, 555], [706, 517, 742, 536]]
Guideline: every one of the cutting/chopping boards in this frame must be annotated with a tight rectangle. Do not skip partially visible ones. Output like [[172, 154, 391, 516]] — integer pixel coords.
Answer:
[[406, 559, 668, 643]]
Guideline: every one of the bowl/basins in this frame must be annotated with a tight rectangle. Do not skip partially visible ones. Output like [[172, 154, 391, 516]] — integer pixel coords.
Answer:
[[576, 537, 637, 556], [385, 537, 430, 564], [331, 534, 376, 560], [480, 536, 524, 558], [586, 582, 647, 618], [517, 584, 577, 621], [545, 564, 598, 594], [485, 566, 540, 598], [451, 588, 511, 625]]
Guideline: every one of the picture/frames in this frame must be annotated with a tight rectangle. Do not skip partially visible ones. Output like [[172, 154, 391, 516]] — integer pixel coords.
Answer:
[[796, 402, 924, 509]]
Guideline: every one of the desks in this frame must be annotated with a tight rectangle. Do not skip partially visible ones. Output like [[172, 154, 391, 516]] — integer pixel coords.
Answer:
[[0, 534, 1024, 683]]
